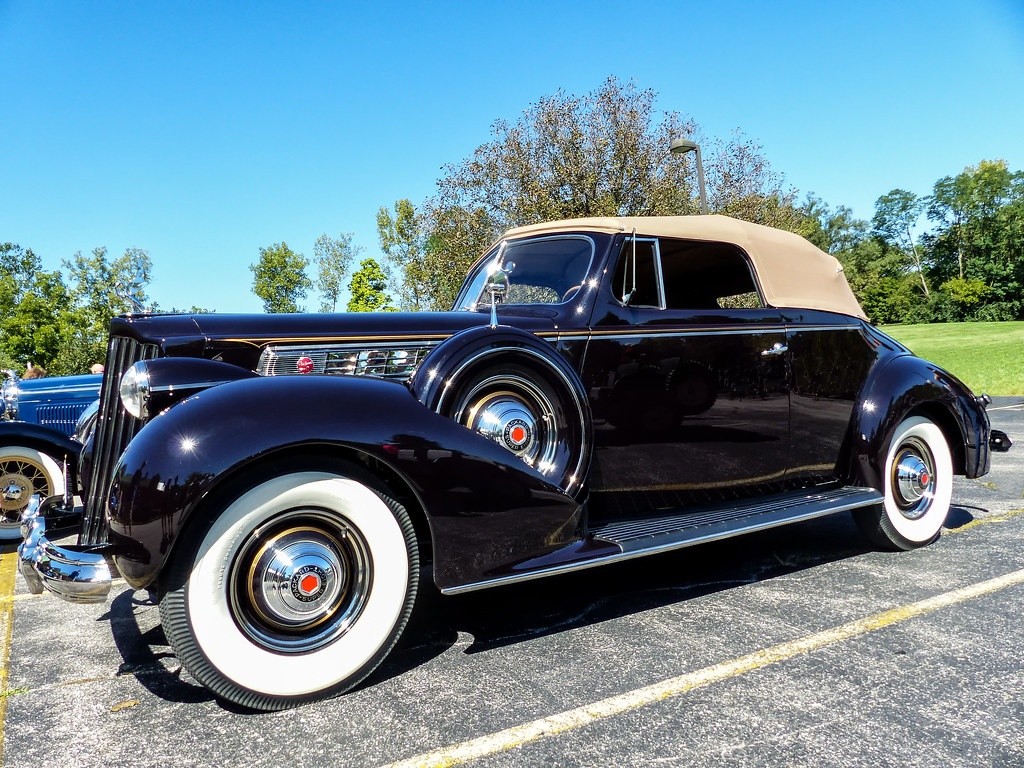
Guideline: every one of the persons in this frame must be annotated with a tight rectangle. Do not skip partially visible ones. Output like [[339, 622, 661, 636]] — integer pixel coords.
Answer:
[[111, 460, 147, 536], [67, 570, 81, 582], [92, 364, 104, 373], [161, 473, 209, 542], [22, 367, 47, 378]]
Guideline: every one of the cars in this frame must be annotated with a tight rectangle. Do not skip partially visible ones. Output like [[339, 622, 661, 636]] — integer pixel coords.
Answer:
[[0, 215, 1012, 712]]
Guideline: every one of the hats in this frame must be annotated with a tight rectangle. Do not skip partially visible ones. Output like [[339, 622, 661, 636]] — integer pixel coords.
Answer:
[[91, 364, 104, 371]]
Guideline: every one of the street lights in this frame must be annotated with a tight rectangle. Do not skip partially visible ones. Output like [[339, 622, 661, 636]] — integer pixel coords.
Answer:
[[671, 139, 708, 216]]
